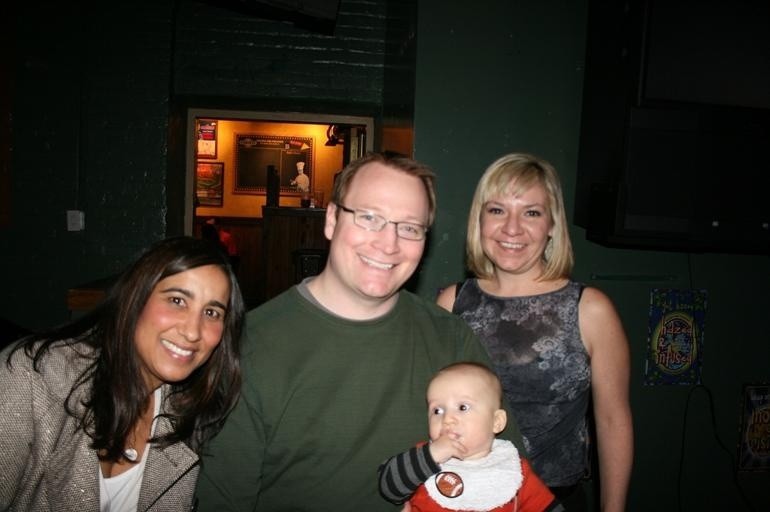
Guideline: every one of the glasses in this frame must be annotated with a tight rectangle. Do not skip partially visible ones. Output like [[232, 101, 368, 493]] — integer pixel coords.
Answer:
[[337, 203, 429, 240]]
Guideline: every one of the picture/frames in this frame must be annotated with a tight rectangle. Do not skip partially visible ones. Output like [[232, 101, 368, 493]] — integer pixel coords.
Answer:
[[196, 119, 218, 159], [194, 161, 224, 208]]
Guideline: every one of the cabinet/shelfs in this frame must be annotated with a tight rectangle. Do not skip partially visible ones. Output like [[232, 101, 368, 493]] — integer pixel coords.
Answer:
[[568, 1, 770, 257], [262, 205, 330, 305]]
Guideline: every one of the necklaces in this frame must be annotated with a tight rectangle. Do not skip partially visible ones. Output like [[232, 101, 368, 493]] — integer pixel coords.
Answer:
[[124, 424, 140, 461]]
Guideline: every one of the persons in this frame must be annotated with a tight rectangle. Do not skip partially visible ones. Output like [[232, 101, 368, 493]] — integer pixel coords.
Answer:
[[433, 152, 637, 510], [376, 362, 567, 512], [288, 169, 309, 192], [189, 149, 528, 512], [0, 235, 249, 512]]
